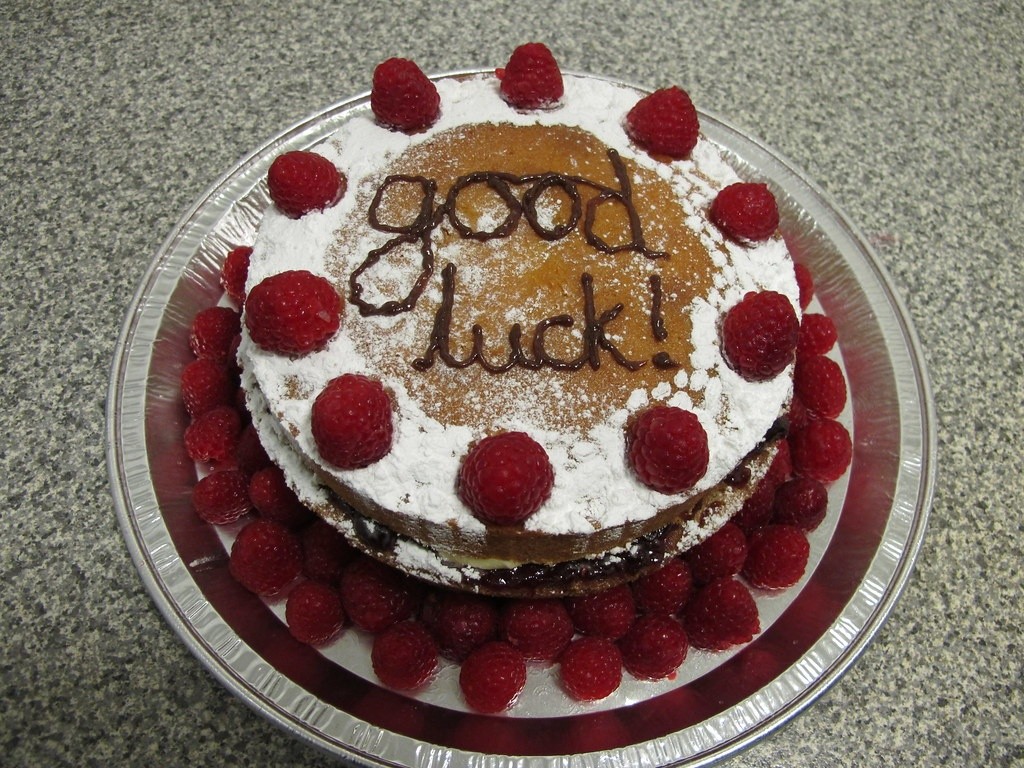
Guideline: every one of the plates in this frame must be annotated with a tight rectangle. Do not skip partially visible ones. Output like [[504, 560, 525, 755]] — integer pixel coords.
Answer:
[[105, 67, 936, 768]]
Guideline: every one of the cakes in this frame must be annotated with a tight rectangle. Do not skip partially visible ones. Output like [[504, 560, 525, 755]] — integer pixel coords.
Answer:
[[183, 40, 853, 714]]
